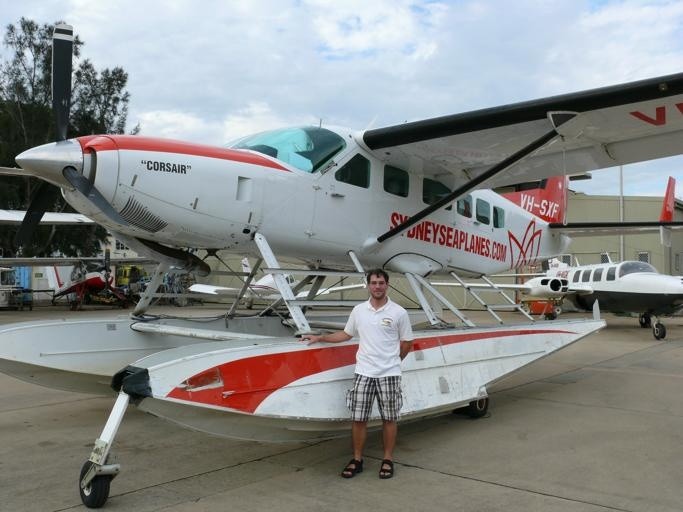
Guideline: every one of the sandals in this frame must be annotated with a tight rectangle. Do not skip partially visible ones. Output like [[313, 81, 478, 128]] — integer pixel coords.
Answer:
[[379, 459, 393, 479], [341, 459, 363, 478]]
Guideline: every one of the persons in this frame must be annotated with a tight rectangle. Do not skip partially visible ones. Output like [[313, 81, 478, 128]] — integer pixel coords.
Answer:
[[297, 268, 414, 479]]
